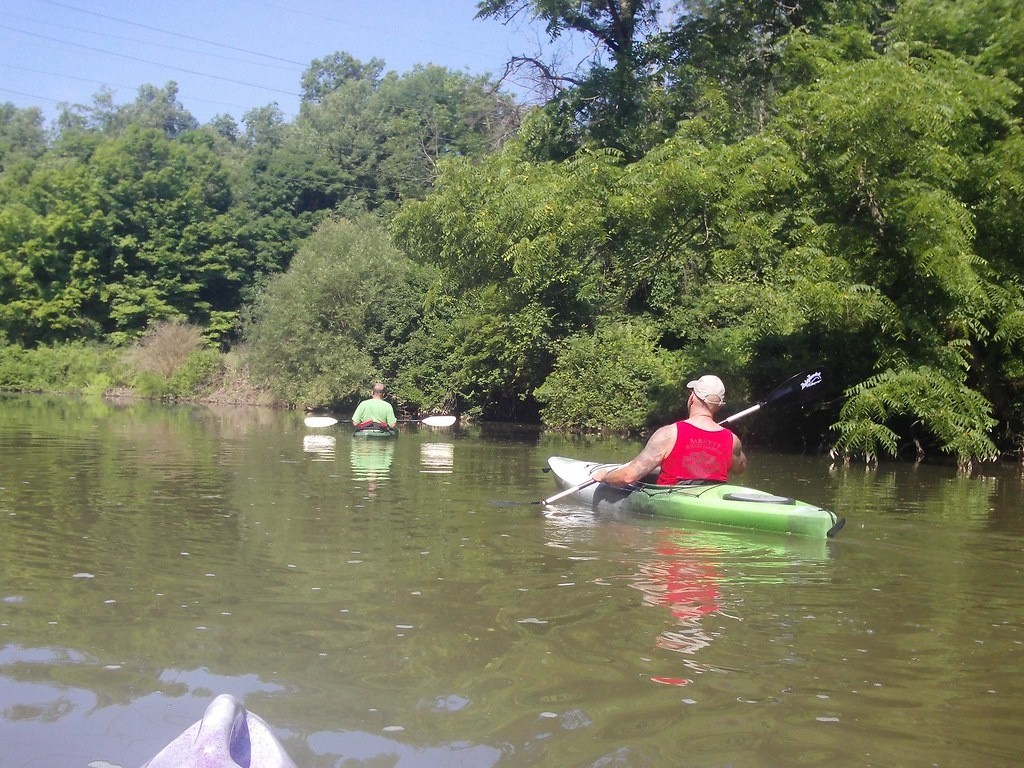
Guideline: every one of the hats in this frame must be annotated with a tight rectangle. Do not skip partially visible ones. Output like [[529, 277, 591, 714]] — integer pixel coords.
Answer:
[[687, 375, 726, 406]]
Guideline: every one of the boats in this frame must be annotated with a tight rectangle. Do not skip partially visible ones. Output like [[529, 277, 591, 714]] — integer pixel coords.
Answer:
[[547, 454, 848, 536], [86, 693, 293, 768], [351, 414, 399, 442]]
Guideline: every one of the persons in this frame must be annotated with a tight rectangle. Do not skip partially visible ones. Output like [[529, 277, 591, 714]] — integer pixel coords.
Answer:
[[351, 382, 397, 429], [591, 375, 748, 488]]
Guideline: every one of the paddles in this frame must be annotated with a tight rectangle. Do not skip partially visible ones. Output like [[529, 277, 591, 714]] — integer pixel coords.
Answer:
[[303, 415, 458, 428], [531, 364, 835, 506]]
